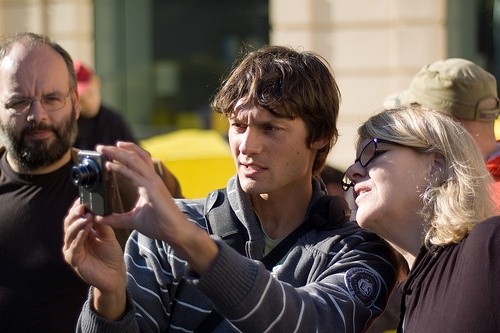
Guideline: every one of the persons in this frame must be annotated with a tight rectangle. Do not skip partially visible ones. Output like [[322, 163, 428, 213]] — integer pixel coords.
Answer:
[[0, 31, 181, 333], [383, 57, 500, 217], [73, 63, 136, 151], [62, 44, 396, 333], [340, 106, 500, 333]]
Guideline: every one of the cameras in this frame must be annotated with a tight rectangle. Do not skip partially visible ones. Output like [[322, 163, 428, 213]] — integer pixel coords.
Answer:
[[69, 150, 113, 217]]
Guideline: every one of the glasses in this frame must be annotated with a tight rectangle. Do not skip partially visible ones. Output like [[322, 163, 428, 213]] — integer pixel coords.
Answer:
[[341, 137, 417, 192], [1, 85, 75, 115]]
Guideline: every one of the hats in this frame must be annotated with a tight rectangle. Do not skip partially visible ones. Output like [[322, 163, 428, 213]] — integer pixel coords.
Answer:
[[384, 58, 500, 122], [74, 62, 92, 95]]
